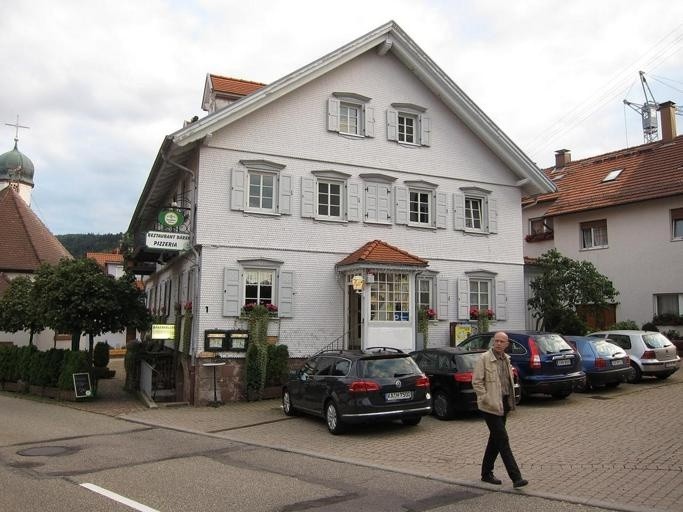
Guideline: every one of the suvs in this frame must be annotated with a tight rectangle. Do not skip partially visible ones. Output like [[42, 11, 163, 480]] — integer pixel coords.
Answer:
[[457, 332, 587, 399], [281, 347, 434, 434]]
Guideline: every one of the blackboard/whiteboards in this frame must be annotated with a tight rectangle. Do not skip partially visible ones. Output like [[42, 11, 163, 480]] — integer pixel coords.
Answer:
[[73, 373, 92, 398]]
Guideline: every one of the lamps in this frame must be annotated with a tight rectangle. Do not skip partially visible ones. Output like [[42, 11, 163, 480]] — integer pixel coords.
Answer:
[[351, 275, 366, 294]]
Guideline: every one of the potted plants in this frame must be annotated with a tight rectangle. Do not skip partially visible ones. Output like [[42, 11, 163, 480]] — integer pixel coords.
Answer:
[[245, 342, 290, 402], [0, 340, 97, 401]]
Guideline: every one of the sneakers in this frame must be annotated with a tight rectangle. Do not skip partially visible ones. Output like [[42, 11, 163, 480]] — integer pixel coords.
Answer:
[[480, 476, 502, 485], [513, 479, 529, 487]]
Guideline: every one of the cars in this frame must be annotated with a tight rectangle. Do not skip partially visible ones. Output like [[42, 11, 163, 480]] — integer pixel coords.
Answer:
[[409, 347, 521, 419], [585, 330, 681, 383], [564, 336, 633, 393]]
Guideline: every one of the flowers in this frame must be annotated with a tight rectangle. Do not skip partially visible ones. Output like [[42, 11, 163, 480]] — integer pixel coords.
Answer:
[[240, 301, 278, 314], [424, 307, 494, 319]]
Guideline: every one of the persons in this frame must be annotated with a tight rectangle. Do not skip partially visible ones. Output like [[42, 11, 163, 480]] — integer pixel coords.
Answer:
[[470, 330, 531, 489]]
